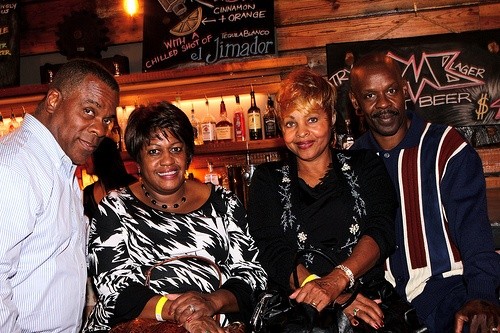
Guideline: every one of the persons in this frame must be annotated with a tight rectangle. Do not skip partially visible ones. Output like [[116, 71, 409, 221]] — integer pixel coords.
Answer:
[[82, 137, 137, 322], [0, 59, 120, 333], [347, 54, 500, 333], [246, 69, 398, 333], [82, 100, 269, 333]]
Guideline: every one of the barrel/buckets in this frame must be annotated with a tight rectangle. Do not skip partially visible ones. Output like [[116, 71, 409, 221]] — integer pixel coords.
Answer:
[[227, 165, 257, 228]]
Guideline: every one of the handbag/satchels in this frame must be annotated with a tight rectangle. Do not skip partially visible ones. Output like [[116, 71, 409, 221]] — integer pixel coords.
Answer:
[[245, 249, 360, 333], [109, 255, 244, 333]]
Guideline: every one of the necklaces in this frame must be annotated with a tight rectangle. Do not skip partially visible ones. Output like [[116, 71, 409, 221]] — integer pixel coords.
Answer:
[[141, 184, 186, 208]]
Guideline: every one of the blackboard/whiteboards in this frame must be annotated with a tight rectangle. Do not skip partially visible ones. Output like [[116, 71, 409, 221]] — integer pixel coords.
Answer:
[[326, 28, 500, 149], [0, 0, 21, 88], [142, 0, 276, 72]]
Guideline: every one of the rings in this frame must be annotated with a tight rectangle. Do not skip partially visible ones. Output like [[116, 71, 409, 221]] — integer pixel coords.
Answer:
[[187, 304, 195, 313], [354, 308, 359, 316], [312, 303, 316, 307]]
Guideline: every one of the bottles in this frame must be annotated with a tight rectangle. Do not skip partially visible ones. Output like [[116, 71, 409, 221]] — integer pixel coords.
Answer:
[[247, 83, 263, 140], [0, 104, 28, 142], [263, 94, 278, 139], [205, 161, 216, 174], [101, 104, 136, 151], [215, 96, 233, 142], [232, 94, 246, 142], [201, 96, 216, 142], [189, 104, 203, 145]]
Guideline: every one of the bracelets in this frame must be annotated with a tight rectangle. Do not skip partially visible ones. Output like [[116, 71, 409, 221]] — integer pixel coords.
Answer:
[[335, 265, 355, 289], [155, 296, 168, 321], [301, 274, 321, 287]]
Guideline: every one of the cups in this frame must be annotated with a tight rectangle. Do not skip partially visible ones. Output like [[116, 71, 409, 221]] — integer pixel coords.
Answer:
[[187, 173, 194, 180], [205, 175, 218, 185]]
